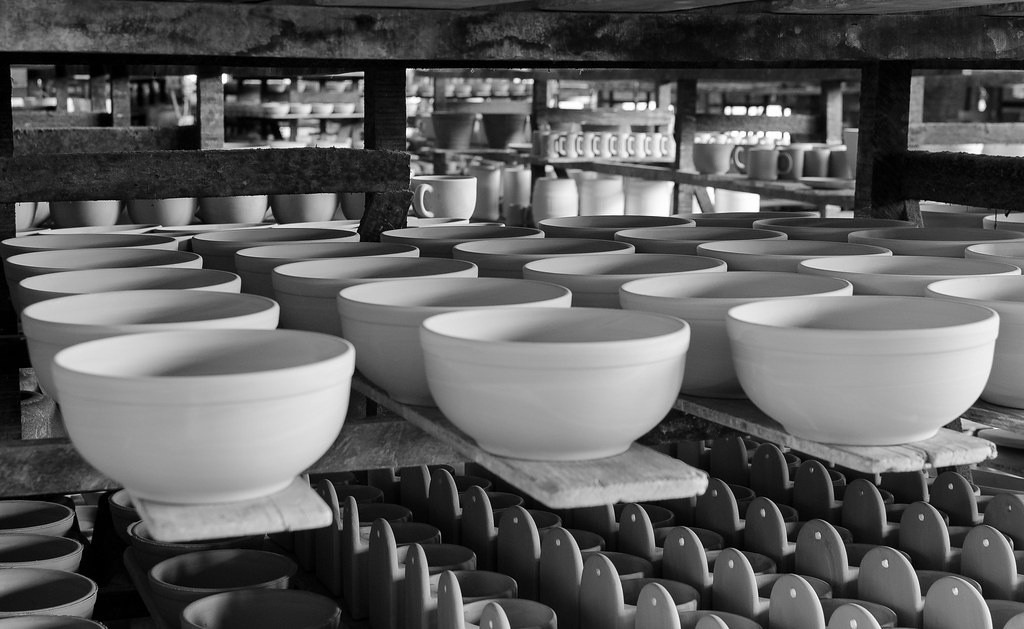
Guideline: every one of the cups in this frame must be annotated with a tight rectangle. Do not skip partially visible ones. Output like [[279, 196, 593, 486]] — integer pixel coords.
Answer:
[[411, 133, 849, 220]]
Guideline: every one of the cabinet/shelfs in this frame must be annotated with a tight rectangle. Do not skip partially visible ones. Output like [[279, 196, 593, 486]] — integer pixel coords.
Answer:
[[11, 63, 1024, 214]]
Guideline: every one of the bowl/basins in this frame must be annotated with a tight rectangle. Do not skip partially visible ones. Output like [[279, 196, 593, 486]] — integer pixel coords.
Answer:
[[3, 77, 1023, 629]]
